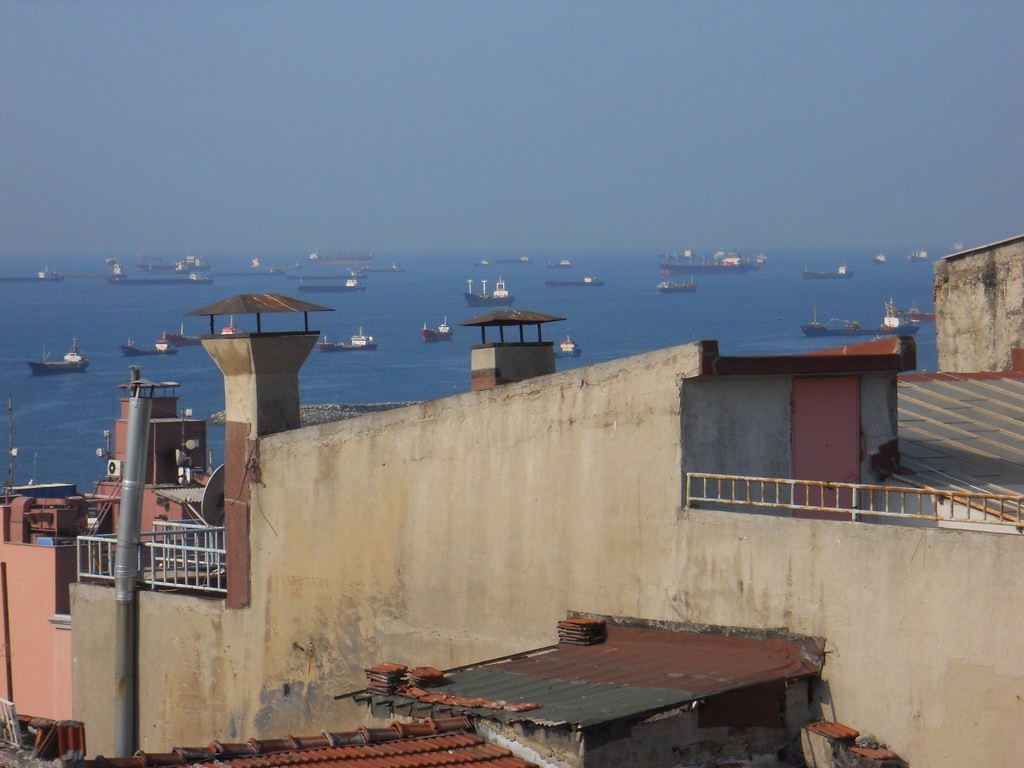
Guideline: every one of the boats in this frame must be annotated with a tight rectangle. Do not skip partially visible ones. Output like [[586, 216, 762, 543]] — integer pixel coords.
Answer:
[[249, 259, 306, 273], [544, 276, 604, 289], [547, 260, 572, 267], [117, 330, 180, 357], [473, 258, 492, 267], [132, 248, 210, 273], [309, 249, 374, 266], [189, 273, 213, 282], [555, 333, 583, 358], [164, 315, 245, 345], [296, 269, 367, 291], [28, 335, 91, 377], [316, 327, 378, 352], [113, 264, 128, 279], [518, 255, 531, 263], [658, 239, 964, 338], [105, 257, 119, 269], [462, 275, 516, 309], [360, 258, 407, 272], [421, 316, 454, 344], [37, 263, 66, 282], [286, 265, 368, 279]]
